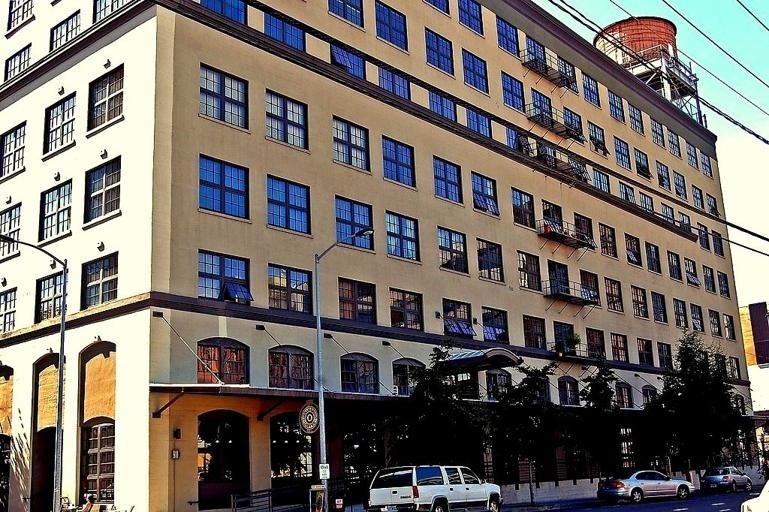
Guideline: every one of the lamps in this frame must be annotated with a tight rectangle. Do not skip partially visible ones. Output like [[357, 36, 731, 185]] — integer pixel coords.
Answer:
[[1, 449, 11, 465]]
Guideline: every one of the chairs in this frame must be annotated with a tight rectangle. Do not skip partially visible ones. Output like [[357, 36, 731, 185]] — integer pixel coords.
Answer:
[[58, 497, 69, 512], [87, 504, 107, 512]]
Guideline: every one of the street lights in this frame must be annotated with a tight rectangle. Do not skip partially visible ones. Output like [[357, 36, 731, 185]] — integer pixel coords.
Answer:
[[313, 226, 374, 511], [0, 234, 67, 511]]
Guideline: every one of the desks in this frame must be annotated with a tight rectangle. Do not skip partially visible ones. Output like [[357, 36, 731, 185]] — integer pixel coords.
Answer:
[[62, 505, 79, 512]]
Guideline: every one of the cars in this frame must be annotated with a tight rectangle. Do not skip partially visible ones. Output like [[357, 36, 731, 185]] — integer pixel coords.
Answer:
[[597, 469, 695, 502], [701, 465, 753, 492]]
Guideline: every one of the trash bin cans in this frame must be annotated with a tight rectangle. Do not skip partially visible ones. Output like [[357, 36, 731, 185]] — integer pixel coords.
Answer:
[[686, 470, 700, 490], [328, 495, 345, 512]]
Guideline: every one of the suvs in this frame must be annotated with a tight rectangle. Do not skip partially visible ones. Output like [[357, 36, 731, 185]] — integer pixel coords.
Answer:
[[368, 465, 504, 512]]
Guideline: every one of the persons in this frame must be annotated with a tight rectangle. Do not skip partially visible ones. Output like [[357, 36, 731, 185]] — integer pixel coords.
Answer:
[[77, 495, 95, 512]]
